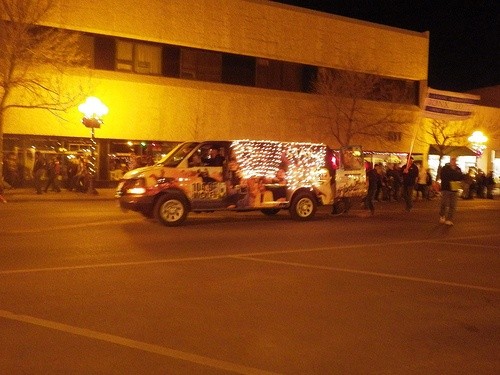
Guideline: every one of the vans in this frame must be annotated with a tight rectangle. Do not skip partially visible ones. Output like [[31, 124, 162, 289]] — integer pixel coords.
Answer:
[[116, 140, 369, 225]]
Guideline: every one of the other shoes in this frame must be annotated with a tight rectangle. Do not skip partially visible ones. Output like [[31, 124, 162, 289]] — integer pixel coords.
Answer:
[[405, 205, 411, 210], [43, 190, 47, 193], [330, 210, 344, 217], [344, 204, 352, 213], [57, 190, 62, 192], [445, 220, 453, 226], [440, 216, 445, 223], [35, 192, 42, 195]]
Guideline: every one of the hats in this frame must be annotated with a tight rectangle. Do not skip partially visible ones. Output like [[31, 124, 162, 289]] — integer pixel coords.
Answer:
[[450, 156, 456, 161]]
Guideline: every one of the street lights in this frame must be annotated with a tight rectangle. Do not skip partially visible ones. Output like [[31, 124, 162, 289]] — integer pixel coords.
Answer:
[[78, 94, 110, 195], [468, 130, 489, 169]]
